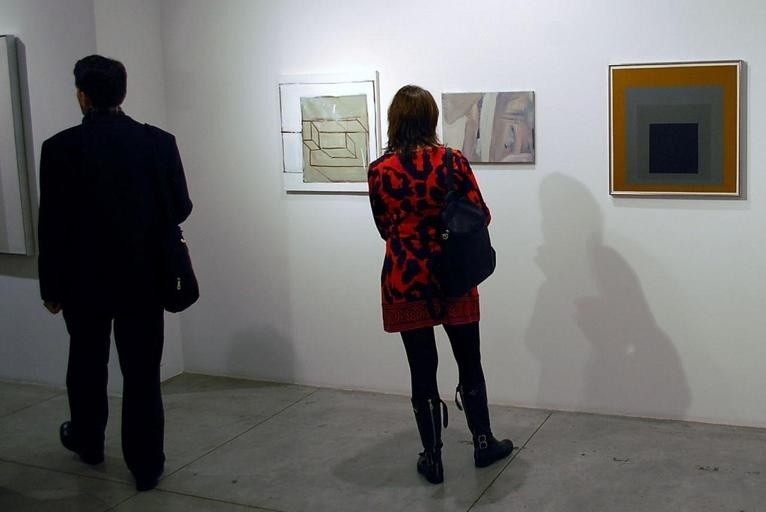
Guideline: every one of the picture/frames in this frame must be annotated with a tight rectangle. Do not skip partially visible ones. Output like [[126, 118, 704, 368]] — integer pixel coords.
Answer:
[[607, 58, 743, 199]]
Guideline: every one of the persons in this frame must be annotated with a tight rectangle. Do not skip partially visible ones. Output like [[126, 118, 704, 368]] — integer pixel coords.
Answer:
[[366, 84, 514, 486], [37, 56, 195, 494]]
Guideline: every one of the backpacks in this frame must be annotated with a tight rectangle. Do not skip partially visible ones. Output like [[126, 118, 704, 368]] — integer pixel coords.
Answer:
[[423, 145, 496, 321]]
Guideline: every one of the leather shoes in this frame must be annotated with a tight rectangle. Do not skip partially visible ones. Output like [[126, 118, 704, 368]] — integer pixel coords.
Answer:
[[127, 451, 165, 492], [56, 419, 106, 466]]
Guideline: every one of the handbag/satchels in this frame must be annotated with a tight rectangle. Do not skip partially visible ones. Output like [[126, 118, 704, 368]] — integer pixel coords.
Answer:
[[141, 226, 200, 315]]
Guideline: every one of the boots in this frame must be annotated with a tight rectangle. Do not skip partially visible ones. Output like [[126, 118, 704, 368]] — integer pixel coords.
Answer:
[[411, 387, 448, 485], [454, 383, 513, 469]]
